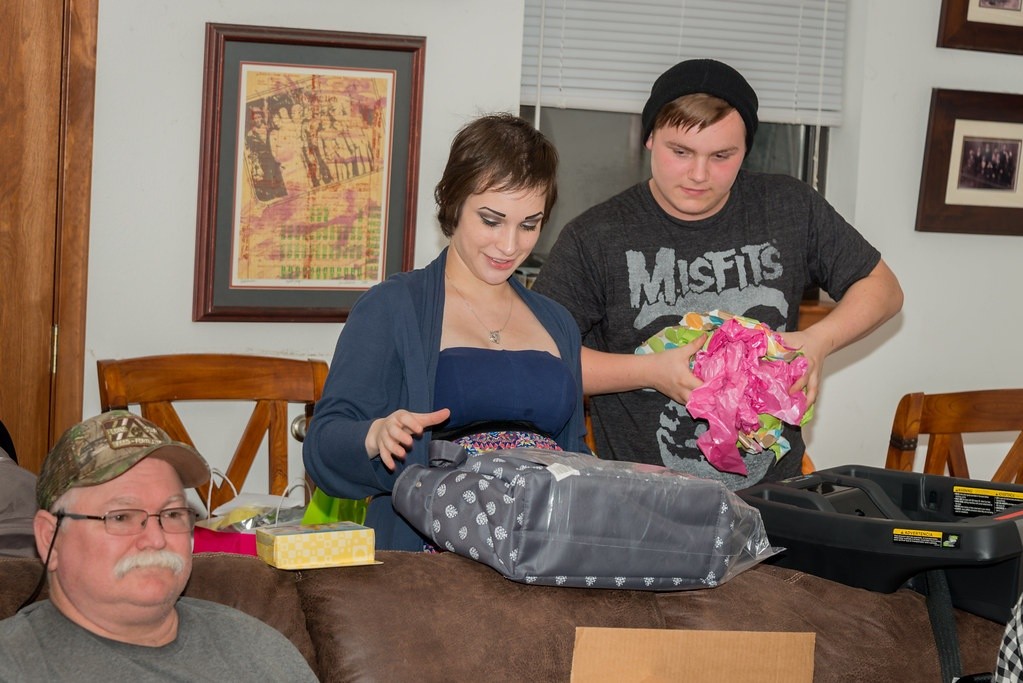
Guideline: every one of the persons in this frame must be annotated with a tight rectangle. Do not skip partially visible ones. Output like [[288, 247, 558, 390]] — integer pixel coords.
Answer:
[[960, 137, 1020, 189], [239, 79, 383, 202], [1, 406, 319, 683], [531, 58, 906, 500], [301, 107, 598, 554]]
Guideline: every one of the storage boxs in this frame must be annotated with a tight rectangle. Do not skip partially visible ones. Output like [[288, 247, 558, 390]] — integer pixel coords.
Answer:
[[254, 518, 383, 569]]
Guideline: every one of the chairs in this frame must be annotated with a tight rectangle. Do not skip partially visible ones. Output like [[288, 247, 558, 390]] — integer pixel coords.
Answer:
[[99, 352, 331, 518], [885, 386, 1023, 486]]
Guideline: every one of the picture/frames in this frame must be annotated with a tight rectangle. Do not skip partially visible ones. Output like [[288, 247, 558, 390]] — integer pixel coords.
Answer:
[[916, 87, 1023, 237], [937, 1, 1023, 56], [191, 21, 428, 321]]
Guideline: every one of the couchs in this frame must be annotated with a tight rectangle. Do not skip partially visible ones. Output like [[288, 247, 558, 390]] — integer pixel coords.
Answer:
[[2, 549, 1005, 682]]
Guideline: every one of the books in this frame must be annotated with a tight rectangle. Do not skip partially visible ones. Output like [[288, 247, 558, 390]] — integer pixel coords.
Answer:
[[249, 517, 379, 571]]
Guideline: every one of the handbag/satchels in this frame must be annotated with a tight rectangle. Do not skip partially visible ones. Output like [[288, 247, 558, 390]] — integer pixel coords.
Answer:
[[301, 484, 368, 528], [189, 466, 315, 557]]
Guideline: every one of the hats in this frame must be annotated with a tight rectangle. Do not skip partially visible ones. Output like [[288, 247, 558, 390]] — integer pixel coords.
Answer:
[[34, 408, 211, 514], [640, 59, 759, 159]]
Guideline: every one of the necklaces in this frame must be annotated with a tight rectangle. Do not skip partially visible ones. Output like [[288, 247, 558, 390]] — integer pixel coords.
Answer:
[[444, 270, 515, 344]]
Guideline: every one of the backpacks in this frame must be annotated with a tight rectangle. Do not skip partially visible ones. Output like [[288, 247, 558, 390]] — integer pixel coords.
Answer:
[[389, 438, 783, 592]]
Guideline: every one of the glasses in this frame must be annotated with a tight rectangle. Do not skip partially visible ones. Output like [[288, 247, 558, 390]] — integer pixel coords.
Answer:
[[51, 505, 200, 536]]
